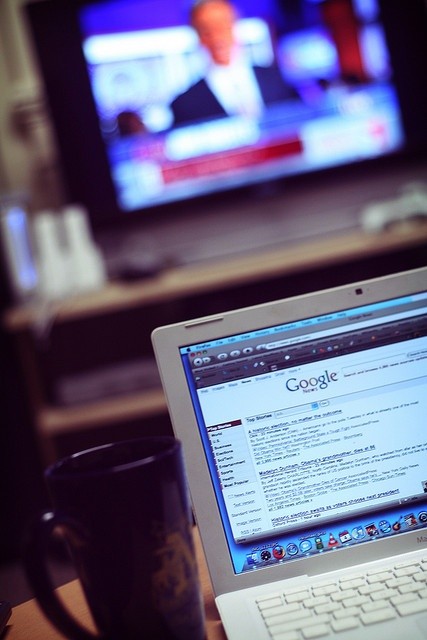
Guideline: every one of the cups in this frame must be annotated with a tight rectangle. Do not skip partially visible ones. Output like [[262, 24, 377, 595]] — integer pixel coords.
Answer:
[[18, 437, 209, 640]]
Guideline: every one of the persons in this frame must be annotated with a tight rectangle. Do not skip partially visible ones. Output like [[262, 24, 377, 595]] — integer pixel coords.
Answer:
[[169, 0, 298, 124]]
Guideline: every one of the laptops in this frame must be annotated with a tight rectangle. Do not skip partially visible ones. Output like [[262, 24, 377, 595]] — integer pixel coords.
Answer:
[[149, 265, 427, 640]]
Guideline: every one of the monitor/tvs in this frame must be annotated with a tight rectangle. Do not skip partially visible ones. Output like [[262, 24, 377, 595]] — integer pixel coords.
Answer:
[[19, 0, 427, 232]]
